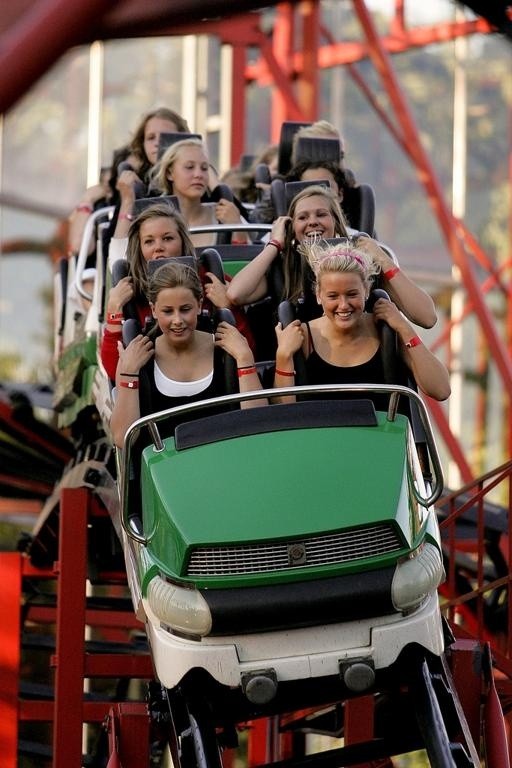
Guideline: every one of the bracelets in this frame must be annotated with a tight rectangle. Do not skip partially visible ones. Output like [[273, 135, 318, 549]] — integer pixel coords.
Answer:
[[266, 239, 282, 253], [121, 373, 140, 388], [107, 312, 125, 325], [238, 367, 256, 376], [383, 266, 400, 282], [275, 369, 296, 376], [404, 334, 422, 349], [236, 365, 255, 369]]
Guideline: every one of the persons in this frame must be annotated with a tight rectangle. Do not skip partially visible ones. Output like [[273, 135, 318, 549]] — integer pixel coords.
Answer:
[[227, 184, 437, 329], [68, 108, 254, 309], [110, 265, 269, 527], [272, 230, 452, 423], [100, 197, 233, 381], [253, 120, 357, 245]]
